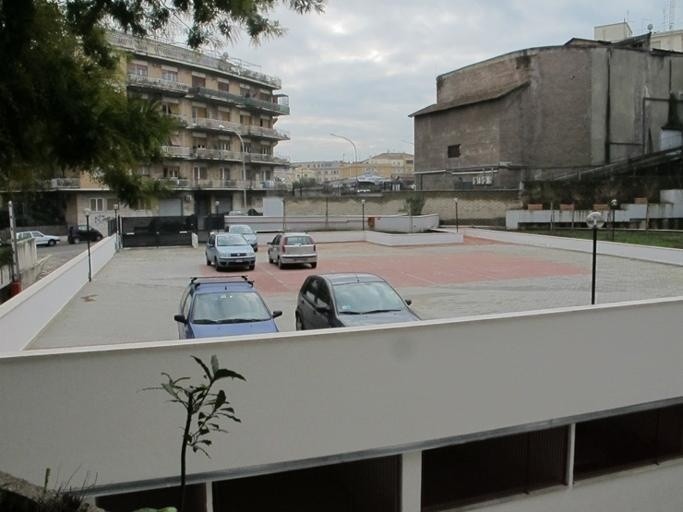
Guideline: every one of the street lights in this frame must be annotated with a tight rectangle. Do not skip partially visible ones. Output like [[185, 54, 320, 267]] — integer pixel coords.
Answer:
[[83, 208, 92, 282], [611, 199, 618, 241], [453, 196, 459, 230], [112, 203, 118, 242], [586, 211, 605, 305], [329, 132, 357, 163]]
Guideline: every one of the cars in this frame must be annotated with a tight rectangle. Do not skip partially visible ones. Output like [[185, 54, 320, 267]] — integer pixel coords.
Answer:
[[15, 230, 61, 246], [68, 225, 103, 244], [205, 224, 318, 271], [174, 271, 423, 340]]
